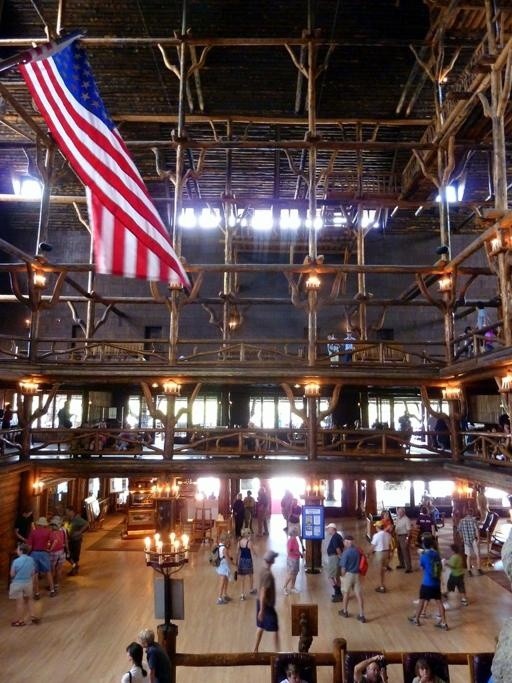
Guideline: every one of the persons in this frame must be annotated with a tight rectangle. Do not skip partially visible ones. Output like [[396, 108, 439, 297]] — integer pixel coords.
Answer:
[[281, 526, 303, 596], [338, 536, 368, 624], [432, 417, 451, 446], [395, 507, 412, 574], [8, 542, 38, 628], [408, 538, 449, 629], [25, 515, 58, 597], [252, 550, 280, 653], [0, 402, 13, 429], [90, 420, 108, 450], [494, 411, 510, 450], [243, 489, 256, 532], [208, 544, 230, 567], [378, 510, 395, 572], [255, 484, 270, 538], [120, 641, 147, 683], [462, 324, 472, 355], [369, 416, 382, 432], [457, 507, 486, 576], [286, 494, 293, 532], [324, 522, 344, 599], [257, 488, 266, 536], [428, 504, 442, 522], [114, 423, 134, 450], [281, 489, 292, 529], [18, 26, 194, 295], [287, 498, 301, 537], [233, 528, 258, 601], [352, 655, 388, 683], [57, 399, 73, 428], [397, 408, 413, 437], [470, 301, 495, 335], [63, 503, 91, 576], [367, 521, 393, 593], [49, 500, 66, 514], [279, 662, 310, 683], [415, 506, 435, 545], [50, 513, 68, 591], [412, 658, 446, 683], [324, 331, 341, 365], [417, 490, 433, 505], [480, 502, 499, 540], [229, 492, 245, 540], [11, 505, 33, 556], [419, 530, 443, 621], [405, 535, 409, 540], [478, 326, 499, 351], [136, 628, 172, 683], [343, 331, 355, 363], [209, 530, 234, 604], [443, 541, 469, 606]]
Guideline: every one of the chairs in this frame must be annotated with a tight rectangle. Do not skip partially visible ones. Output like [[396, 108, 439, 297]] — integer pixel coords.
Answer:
[[193, 506, 214, 547]]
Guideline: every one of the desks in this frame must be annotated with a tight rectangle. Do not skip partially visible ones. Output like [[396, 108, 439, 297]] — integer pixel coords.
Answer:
[[216, 513, 232, 545]]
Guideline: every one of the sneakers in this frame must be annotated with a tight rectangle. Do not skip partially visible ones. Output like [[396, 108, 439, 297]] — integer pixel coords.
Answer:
[[55, 585, 59, 590], [433, 614, 442, 621], [435, 624, 447, 630], [11, 621, 24, 626], [374, 587, 384, 592], [332, 594, 340, 597], [409, 618, 419, 625], [459, 599, 467, 606], [47, 586, 53, 590], [31, 617, 40, 622], [224, 596, 233, 600], [413, 600, 419, 604], [386, 567, 392, 571], [289, 589, 298, 594], [50, 591, 56, 597], [250, 589, 257, 594], [356, 614, 364, 623], [416, 613, 426, 618], [467, 572, 471, 577], [215, 599, 226, 604], [338, 610, 348, 617], [33, 594, 40, 599], [241, 594, 246, 601], [70, 572, 75, 575], [477, 570, 481, 576], [442, 595, 448, 600], [281, 589, 288, 595], [332, 599, 341, 602], [75, 570, 79, 573]]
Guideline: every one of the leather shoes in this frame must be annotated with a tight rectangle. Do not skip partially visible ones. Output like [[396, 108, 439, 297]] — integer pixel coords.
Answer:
[[405, 570, 413, 573], [397, 566, 404, 569]]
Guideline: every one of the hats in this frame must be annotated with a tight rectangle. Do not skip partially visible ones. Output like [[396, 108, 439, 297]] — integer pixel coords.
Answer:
[[50, 516, 61, 528], [325, 521, 336, 529], [373, 520, 384, 526], [423, 539, 431, 549], [36, 515, 48, 526], [342, 535, 354, 541], [263, 549, 278, 561]]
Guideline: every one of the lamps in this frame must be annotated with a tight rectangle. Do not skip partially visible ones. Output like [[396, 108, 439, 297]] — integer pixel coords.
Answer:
[[31, 267, 47, 290], [438, 274, 452, 310], [305, 271, 322, 316]]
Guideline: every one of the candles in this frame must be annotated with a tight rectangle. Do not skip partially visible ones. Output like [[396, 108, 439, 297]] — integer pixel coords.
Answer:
[[156, 540, 164, 565], [441, 387, 460, 403], [163, 382, 182, 393], [33, 480, 45, 494], [145, 541, 151, 563], [173, 541, 181, 563], [499, 375, 511, 392], [155, 533, 161, 551], [170, 533, 176, 553], [303, 382, 321, 399], [184, 541, 189, 559], [149, 484, 181, 499], [19, 383, 39, 394], [305, 484, 325, 497]]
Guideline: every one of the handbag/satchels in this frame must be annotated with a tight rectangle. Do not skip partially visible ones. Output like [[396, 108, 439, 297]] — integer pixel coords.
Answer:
[[358, 547, 368, 576]]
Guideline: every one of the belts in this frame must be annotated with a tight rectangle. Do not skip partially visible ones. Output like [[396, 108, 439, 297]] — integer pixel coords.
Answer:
[[33, 550, 47, 552]]
